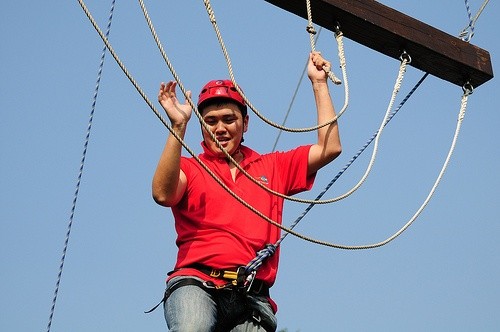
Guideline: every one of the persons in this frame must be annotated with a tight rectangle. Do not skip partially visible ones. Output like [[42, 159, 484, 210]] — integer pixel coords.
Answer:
[[149, 51, 342, 331]]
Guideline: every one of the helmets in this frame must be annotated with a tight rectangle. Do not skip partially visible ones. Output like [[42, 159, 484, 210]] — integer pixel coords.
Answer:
[[197, 80, 247, 114]]
